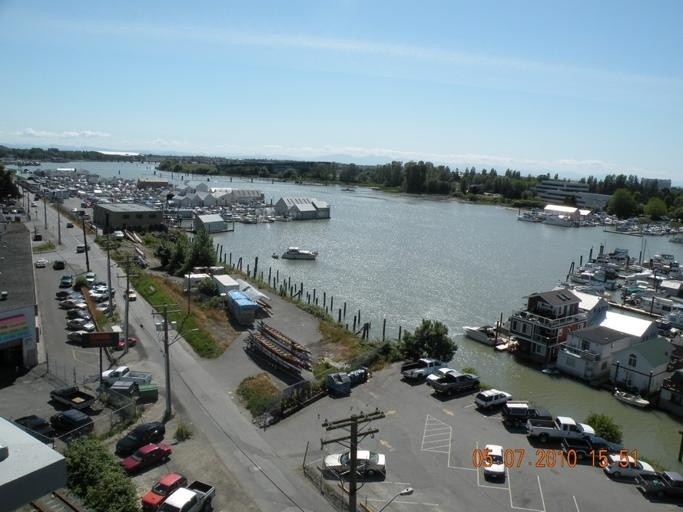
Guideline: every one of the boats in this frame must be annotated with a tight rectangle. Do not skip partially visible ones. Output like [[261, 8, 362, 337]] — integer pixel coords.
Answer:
[[272, 247, 318, 258], [112, 231, 125, 239], [463, 326, 497, 344]]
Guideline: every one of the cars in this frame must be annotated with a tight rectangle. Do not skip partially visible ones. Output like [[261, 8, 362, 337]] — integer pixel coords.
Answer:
[[77, 243, 91, 252], [325, 449, 386, 478], [17, 365, 214, 511], [36, 258, 49, 268], [33, 233, 42, 240], [56, 271, 137, 350], [54, 260, 64, 271]]
